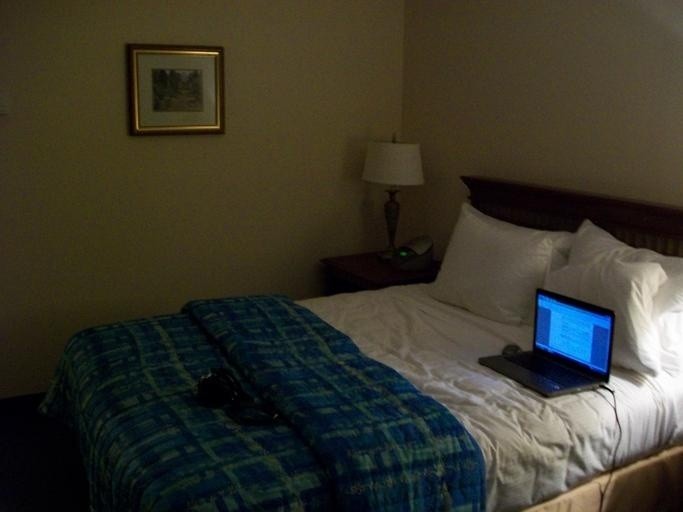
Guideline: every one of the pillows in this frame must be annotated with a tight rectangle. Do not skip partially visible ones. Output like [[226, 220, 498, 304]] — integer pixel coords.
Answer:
[[542, 259, 667, 377], [566, 222, 682, 368], [427, 203, 551, 326]]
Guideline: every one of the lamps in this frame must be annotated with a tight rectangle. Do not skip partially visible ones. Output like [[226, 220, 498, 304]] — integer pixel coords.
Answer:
[[361, 140, 423, 259]]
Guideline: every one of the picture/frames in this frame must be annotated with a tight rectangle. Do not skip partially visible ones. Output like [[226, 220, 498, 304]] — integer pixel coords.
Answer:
[[126, 43, 225, 134]]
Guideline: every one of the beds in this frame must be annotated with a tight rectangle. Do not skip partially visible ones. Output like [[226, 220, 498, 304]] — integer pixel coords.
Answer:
[[37, 175, 682, 511]]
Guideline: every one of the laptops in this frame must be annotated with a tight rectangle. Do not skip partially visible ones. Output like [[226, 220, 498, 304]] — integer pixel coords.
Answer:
[[477, 288, 616, 399]]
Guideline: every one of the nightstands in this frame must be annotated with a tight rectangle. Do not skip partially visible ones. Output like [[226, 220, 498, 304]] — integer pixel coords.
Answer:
[[319, 249, 436, 293]]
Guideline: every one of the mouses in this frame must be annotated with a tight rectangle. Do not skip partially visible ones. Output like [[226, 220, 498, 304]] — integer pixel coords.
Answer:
[[500, 339, 523, 358]]
